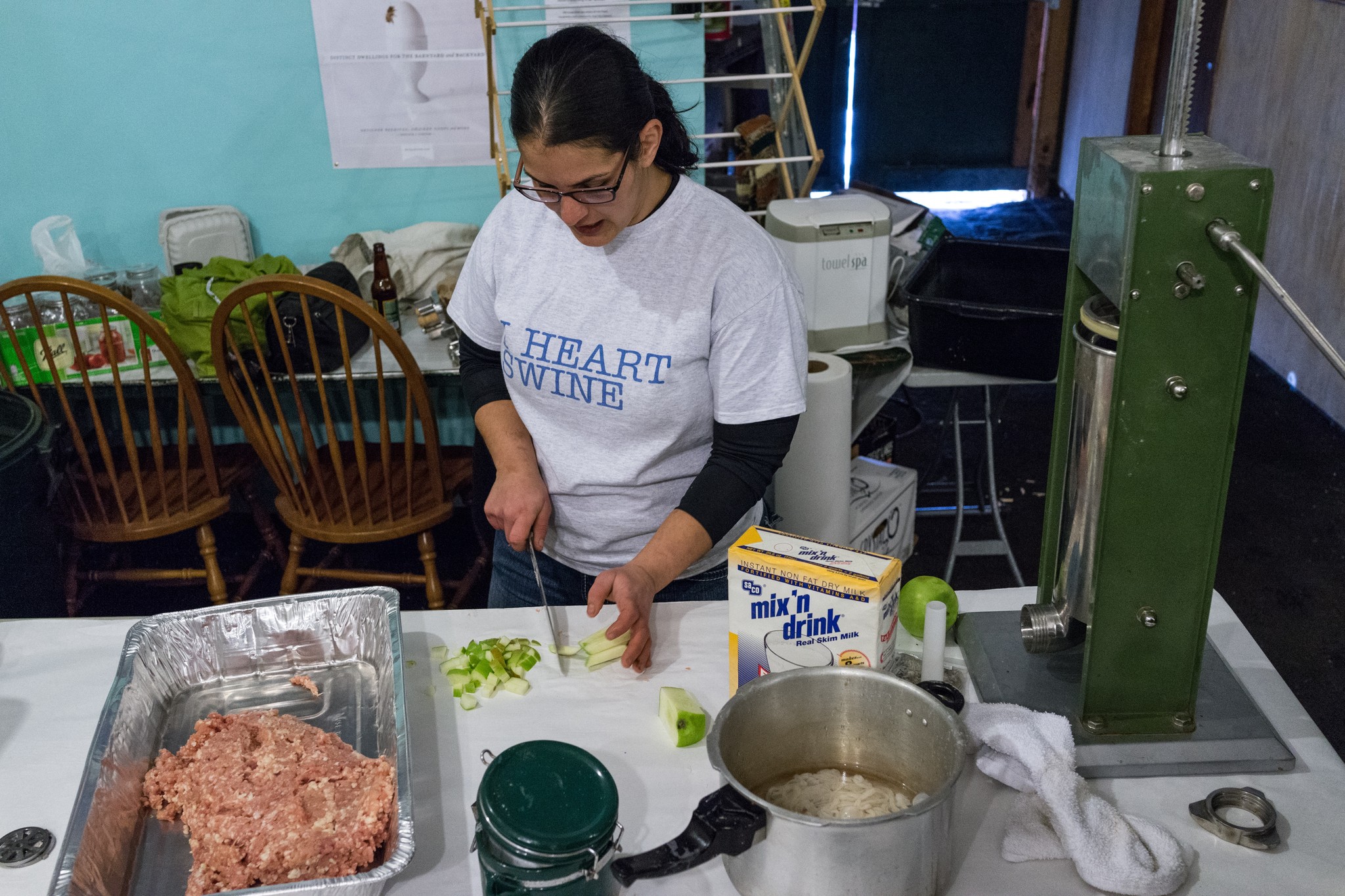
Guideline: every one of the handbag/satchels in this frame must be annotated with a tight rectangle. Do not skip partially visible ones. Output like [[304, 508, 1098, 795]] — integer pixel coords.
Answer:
[[265, 261, 369, 373]]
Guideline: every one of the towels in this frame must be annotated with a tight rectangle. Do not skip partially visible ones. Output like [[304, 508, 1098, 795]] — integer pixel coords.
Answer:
[[964, 699, 1199, 895]]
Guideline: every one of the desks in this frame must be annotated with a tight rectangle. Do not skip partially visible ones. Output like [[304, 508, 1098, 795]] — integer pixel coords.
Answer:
[[0, 586, 1345, 896], [21, 315, 1055, 589]]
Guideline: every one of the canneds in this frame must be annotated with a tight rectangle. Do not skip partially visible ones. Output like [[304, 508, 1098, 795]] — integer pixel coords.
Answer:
[[98, 324, 126, 365]]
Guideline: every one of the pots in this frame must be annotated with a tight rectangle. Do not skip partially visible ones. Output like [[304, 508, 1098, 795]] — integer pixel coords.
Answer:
[[611, 665, 968, 896]]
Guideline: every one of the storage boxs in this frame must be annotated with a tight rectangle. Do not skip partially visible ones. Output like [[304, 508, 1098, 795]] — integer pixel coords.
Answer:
[[729, 527, 901, 698], [851, 414, 920, 570], [0, 303, 172, 384], [898, 236, 1071, 380]]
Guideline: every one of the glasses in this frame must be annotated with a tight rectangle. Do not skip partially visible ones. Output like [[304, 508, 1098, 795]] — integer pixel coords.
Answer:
[[513, 138, 636, 204]]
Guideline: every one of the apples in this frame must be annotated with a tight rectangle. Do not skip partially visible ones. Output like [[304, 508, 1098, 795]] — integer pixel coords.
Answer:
[[407, 635, 542, 710], [546, 622, 631, 666], [899, 575, 958, 638], [658, 687, 707, 747]]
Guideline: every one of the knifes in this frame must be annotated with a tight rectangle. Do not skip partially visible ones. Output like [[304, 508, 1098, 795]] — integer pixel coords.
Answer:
[[525, 527, 566, 675]]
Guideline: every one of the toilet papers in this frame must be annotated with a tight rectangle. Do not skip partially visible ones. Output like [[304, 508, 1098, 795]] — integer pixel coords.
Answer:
[[773, 347, 854, 548]]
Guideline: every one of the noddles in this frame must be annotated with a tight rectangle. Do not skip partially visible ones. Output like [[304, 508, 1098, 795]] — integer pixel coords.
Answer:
[[763, 766, 912, 819]]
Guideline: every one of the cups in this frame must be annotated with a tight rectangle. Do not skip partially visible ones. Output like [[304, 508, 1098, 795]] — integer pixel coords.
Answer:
[[764, 631, 833, 672]]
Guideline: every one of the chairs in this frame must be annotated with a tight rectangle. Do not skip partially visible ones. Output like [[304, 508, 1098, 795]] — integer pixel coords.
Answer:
[[210, 276, 490, 610], [1, 276, 293, 619]]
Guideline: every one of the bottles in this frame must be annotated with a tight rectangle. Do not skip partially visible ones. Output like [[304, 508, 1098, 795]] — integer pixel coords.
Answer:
[[83, 267, 122, 318], [468, 740, 625, 896], [0, 293, 36, 331], [99, 324, 125, 364], [39, 291, 88, 324], [371, 242, 402, 338], [120, 264, 163, 310]]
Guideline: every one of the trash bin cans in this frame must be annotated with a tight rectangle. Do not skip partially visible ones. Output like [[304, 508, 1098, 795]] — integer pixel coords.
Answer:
[[0, 389, 60, 618]]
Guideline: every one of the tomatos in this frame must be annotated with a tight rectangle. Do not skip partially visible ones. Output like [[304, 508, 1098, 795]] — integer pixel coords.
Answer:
[[70, 354, 108, 371]]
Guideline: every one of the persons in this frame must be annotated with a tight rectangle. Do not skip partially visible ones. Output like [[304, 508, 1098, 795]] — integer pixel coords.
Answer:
[[447, 26, 809, 674]]
